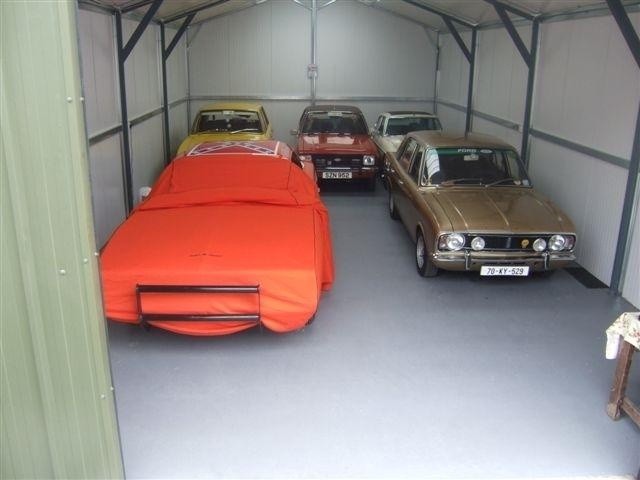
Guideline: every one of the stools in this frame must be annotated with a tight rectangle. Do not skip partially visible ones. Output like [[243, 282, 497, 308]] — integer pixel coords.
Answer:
[[605, 310, 640, 431]]
